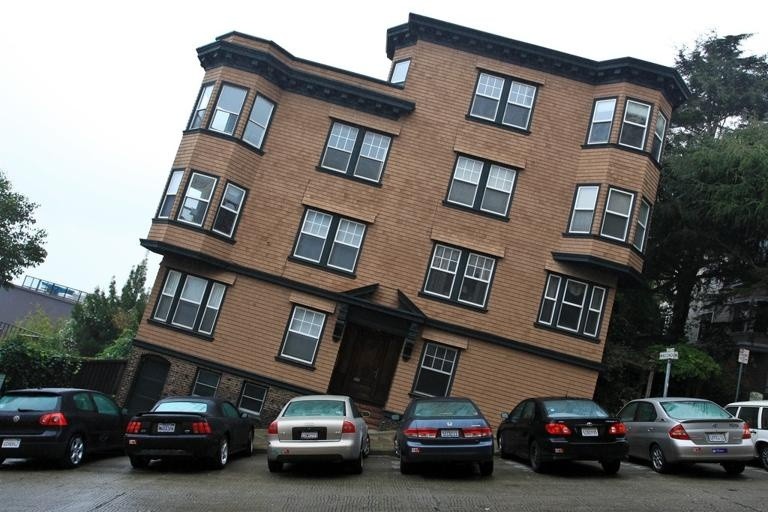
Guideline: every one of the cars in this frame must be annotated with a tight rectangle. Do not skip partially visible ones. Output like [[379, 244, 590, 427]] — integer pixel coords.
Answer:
[[392, 395, 494, 475], [616, 396, 759, 476], [265, 395, 370, 474], [123, 395, 255, 470], [496, 398, 629, 476]]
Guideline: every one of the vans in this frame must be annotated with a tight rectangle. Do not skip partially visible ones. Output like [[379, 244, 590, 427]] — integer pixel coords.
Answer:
[[720, 401, 768, 468]]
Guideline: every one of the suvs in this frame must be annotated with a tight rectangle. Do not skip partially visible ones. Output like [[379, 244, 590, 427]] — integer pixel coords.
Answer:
[[0, 387, 130, 466]]
